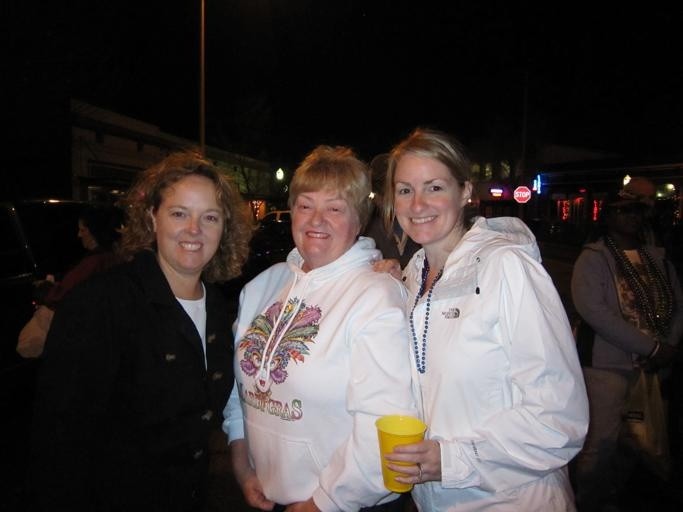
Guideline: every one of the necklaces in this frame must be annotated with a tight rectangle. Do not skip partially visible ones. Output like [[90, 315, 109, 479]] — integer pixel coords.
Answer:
[[410, 264, 444, 373]]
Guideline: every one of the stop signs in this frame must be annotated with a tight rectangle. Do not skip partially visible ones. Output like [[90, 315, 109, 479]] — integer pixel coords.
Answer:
[[514, 186, 532, 204]]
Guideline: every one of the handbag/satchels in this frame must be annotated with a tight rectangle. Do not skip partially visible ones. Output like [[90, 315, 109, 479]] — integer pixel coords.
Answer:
[[16, 305, 55, 358]]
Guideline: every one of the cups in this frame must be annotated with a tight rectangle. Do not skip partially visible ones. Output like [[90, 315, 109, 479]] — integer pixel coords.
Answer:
[[375, 416, 425, 493]]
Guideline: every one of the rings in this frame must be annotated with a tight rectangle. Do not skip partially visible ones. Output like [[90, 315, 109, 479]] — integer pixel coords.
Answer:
[[417, 462, 425, 473], [418, 470, 423, 478]]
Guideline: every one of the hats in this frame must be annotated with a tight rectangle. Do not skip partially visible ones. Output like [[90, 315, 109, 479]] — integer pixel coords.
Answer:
[[607, 175, 657, 207]]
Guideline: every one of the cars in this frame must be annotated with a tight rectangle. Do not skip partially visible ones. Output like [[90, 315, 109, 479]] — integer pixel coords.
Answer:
[[1, 198, 121, 310], [260, 210, 291, 224]]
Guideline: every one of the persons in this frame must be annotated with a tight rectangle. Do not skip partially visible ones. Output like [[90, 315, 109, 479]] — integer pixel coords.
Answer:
[[39, 206, 115, 312], [370, 129, 590, 511], [571, 172, 681, 501], [36, 151, 256, 507], [220, 145, 417, 512]]
